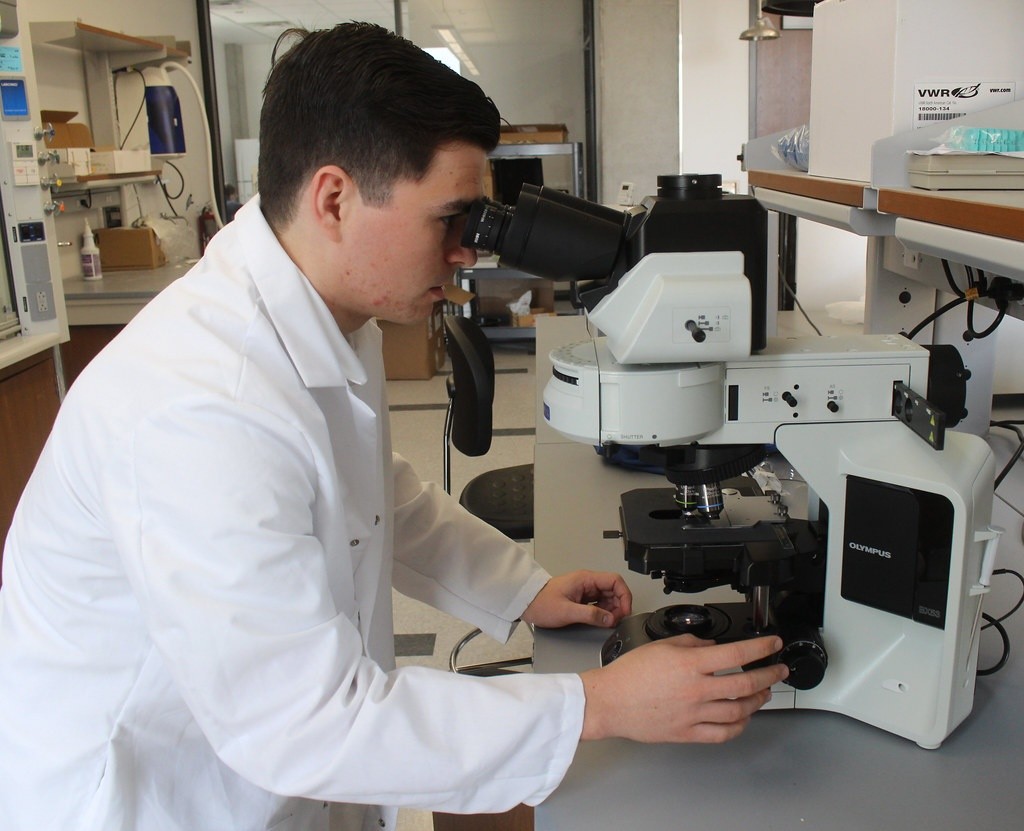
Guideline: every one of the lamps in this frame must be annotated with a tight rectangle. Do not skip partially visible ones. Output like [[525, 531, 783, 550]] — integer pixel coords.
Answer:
[[140, 61, 225, 229], [738, 0, 780, 42]]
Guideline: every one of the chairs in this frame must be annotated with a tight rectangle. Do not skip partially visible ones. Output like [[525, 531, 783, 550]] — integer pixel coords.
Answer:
[[442, 314, 535, 677]]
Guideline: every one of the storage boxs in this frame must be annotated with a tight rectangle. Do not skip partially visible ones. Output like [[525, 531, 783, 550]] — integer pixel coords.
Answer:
[[499, 123, 568, 145], [907, 155, 1024, 191], [478, 280, 555, 316], [511, 307, 549, 327], [91, 227, 170, 272], [377, 284, 476, 381], [806, 0, 1024, 182], [41, 110, 152, 177]]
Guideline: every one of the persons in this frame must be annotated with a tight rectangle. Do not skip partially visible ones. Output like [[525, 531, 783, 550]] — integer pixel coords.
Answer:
[[0, 21, 792, 831]]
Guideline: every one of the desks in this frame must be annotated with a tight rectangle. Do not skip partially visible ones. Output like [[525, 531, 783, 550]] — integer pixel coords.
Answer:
[[61, 259, 194, 327], [540, 312, 1024, 831]]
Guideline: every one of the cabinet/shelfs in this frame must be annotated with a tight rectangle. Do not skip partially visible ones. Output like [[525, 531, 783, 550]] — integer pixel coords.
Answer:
[[27, 21, 204, 192], [454, 142, 583, 339]]
